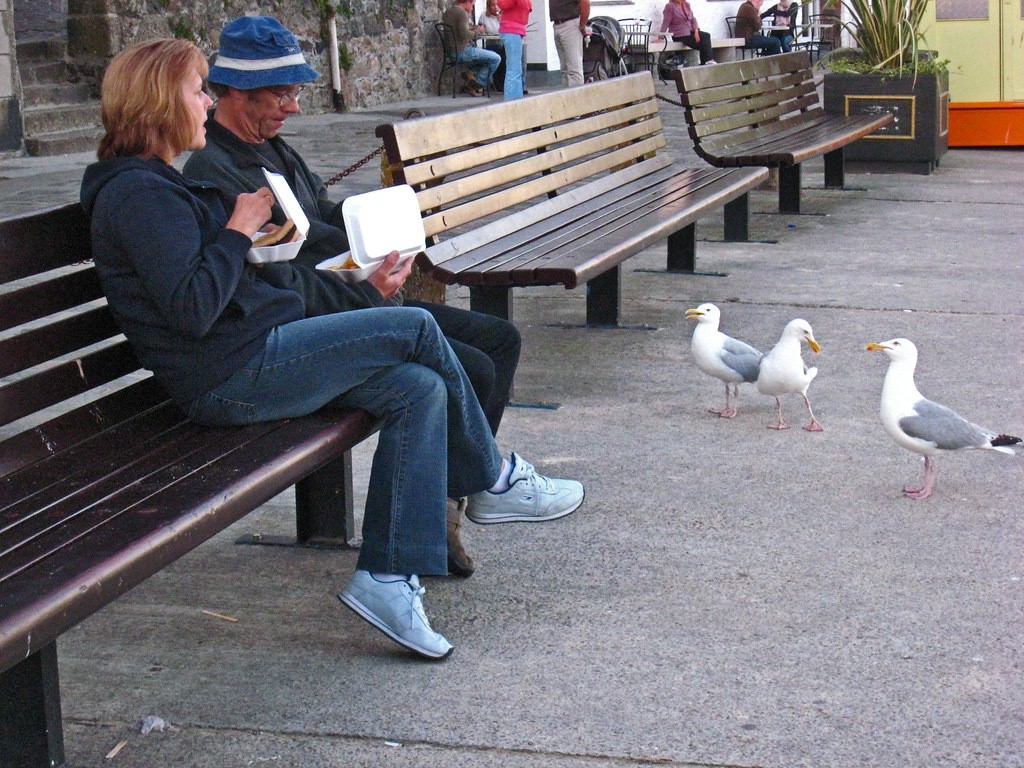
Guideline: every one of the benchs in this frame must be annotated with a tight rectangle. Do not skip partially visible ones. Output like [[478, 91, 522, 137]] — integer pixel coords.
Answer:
[[675, 49, 893, 217], [375, 71, 770, 328], [622, 37, 746, 80], [0, 197, 384, 768]]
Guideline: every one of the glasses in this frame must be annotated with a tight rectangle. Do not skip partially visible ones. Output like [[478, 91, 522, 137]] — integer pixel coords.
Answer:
[[262, 84, 309, 107]]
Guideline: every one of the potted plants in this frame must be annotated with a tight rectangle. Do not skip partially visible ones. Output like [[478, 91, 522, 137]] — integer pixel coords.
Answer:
[[794, 2, 950, 174]]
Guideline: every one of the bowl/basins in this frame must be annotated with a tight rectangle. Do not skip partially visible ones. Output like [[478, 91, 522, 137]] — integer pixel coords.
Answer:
[[315, 184, 426, 285], [245, 167, 310, 264]]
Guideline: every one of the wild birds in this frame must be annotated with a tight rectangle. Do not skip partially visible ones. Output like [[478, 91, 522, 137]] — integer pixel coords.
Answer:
[[864, 337, 1024, 502], [670, 303, 825, 433]]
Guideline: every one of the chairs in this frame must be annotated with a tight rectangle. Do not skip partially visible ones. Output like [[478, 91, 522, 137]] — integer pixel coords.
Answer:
[[725, 14, 840, 62], [617, 18, 655, 78], [434, 23, 492, 99]]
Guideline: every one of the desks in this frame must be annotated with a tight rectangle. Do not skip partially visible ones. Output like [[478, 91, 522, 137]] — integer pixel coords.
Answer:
[[797, 24, 834, 70], [758, 26, 790, 56], [474, 35, 504, 92], [625, 31, 668, 87]]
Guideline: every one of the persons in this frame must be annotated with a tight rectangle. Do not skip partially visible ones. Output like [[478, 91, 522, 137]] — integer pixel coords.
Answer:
[[478, 0, 532, 98], [735, 0, 781, 56], [442, 0, 502, 97], [181, 17, 521, 577], [80, 38, 585, 663], [549, 0, 592, 119], [760, 0, 798, 55], [650, 0, 718, 65]]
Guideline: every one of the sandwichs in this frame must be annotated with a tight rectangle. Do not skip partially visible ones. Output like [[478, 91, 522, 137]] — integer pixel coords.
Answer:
[[251, 218, 299, 247]]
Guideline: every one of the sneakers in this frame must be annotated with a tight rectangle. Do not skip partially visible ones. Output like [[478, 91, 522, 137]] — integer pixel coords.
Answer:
[[465, 451, 585, 525], [336, 569, 455, 661]]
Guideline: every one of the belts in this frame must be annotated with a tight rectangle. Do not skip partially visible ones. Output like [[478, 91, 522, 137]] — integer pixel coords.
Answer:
[[554, 16, 579, 24]]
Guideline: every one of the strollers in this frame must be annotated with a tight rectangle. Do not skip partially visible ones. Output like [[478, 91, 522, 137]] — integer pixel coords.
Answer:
[[582, 14, 633, 85]]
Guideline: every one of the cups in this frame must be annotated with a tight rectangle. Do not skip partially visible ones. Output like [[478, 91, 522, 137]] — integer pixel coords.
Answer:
[[495, 8, 502, 24], [479, 15, 487, 35]]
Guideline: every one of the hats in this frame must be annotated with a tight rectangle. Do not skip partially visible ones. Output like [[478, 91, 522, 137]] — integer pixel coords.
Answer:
[[206, 16, 322, 91]]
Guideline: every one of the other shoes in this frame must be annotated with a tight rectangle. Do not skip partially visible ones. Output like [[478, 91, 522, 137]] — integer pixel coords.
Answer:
[[467, 87, 482, 97], [461, 71, 481, 86], [445, 497, 476, 577], [705, 60, 717, 65]]
